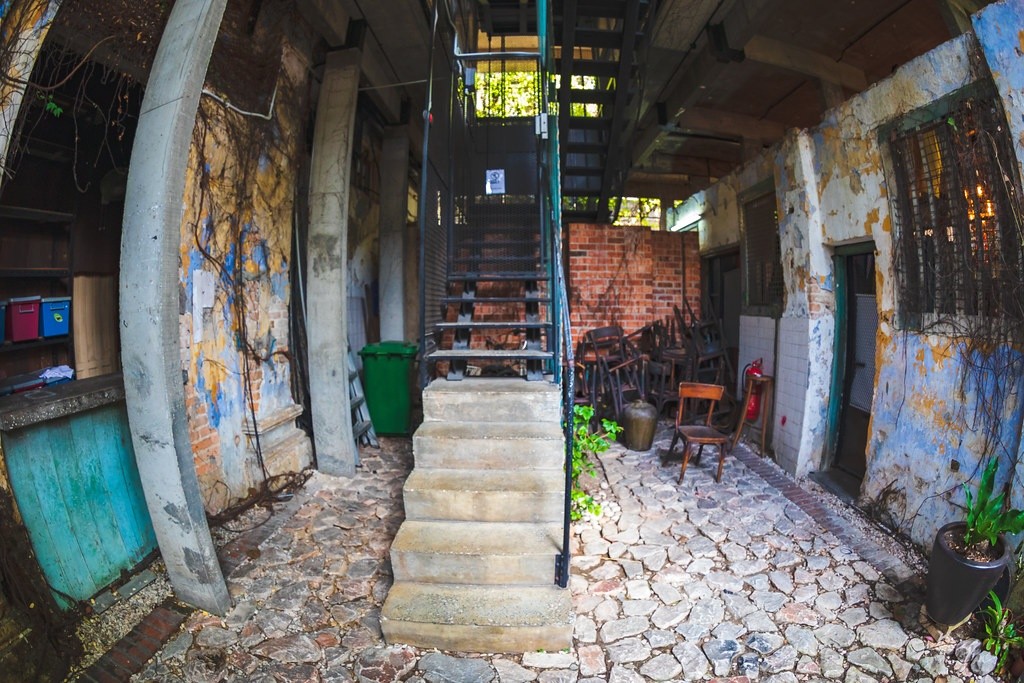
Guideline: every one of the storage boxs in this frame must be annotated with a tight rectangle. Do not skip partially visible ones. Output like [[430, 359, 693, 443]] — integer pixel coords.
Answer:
[[40, 298, 70, 336], [5, 296, 41, 342], [1, 303, 7, 344]]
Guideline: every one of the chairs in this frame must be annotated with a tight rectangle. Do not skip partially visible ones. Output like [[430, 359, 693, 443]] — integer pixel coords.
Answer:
[[663, 383, 731, 485], [566, 294, 742, 434]]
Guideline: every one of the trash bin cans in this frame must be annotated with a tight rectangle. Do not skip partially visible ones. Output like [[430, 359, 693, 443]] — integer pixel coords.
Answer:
[[357, 339, 421, 438]]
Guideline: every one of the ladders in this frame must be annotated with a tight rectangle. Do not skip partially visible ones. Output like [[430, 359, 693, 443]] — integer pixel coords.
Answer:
[[346, 334, 380, 467]]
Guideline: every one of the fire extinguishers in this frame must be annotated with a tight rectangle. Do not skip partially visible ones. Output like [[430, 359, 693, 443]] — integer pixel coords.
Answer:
[[742, 356, 763, 420]]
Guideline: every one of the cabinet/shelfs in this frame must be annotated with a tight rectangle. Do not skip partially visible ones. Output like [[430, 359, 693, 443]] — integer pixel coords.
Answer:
[[1, 136, 77, 397]]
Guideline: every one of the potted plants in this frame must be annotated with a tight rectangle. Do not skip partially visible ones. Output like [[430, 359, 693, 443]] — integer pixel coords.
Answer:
[[975, 591, 1024, 658], [996, 549, 1024, 625], [925, 455, 1024, 626]]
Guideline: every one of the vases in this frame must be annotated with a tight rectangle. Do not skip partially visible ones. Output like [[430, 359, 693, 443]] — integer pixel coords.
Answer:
[[1009, 657, 1024, 681], [1013, 621, 1024, 637]]
